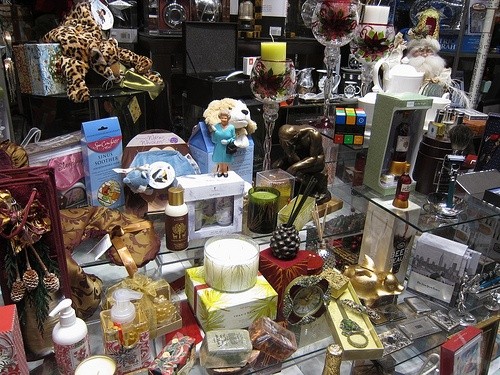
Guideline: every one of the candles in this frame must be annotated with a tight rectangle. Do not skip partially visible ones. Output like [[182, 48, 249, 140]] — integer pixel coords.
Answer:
[[261, 41, 286, 80]]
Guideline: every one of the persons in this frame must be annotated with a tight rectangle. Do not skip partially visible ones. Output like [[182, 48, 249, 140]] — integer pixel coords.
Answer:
[[277, 124, 325, 186], [401, 10, 469, 109], [210, 111, 237, 177]]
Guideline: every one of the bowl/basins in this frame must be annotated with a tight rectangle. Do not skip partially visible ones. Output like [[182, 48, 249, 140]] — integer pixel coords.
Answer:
[[423, 193, 468, 219]]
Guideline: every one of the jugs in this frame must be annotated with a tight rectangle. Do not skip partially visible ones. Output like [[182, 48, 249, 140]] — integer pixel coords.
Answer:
[[293, 66, 315, 94]]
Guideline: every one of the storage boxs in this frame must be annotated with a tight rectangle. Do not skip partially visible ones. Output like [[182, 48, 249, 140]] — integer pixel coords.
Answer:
[[408, 233, 468, 304], [362, 92, 433, 196], [185, 266, 277, 332], [175, 171, 246, 240], [441, 326, 483, 375], [188, 121, 254, 182], [81, 117, 128, 208]]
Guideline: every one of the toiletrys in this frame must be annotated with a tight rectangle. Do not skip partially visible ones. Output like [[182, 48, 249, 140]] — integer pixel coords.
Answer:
[[49, 288, 154, 375]]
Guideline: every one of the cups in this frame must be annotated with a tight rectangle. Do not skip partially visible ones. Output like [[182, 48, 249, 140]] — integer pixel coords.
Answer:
[[247, 187, 281, 235]]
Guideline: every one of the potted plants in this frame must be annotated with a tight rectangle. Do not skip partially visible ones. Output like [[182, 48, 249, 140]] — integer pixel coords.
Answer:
[[6, 241, 70, 354]]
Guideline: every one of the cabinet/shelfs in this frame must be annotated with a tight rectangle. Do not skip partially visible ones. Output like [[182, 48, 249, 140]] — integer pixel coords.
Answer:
[[0, 0, 500, 375]]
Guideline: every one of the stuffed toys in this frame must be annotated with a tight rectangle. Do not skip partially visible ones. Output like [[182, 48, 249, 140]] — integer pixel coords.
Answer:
[[41, 0, 164, 102], [203, 97, 258, 148]]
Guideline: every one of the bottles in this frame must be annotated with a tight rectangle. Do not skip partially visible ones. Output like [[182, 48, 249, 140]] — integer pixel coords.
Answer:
[[216, 195, 232, 227], [395, 163, 412, 201], [391, 112, 414, 162], [164, 188, 189, 251], [193, 199, 202, 231]]
